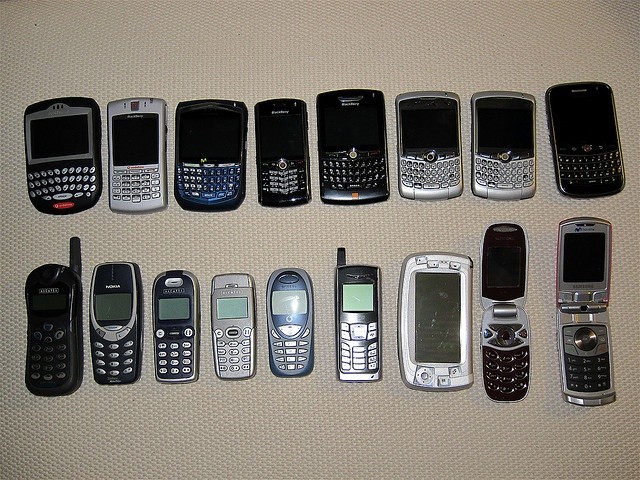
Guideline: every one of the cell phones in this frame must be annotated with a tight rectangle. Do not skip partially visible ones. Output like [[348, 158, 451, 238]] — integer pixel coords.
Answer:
[[254, 98, 314, 209], [545, 81, 625, 199], [25, 236, 83, 398], [471, 90, 537, 200], [22, 97, 102, 216], [90, 262, 142, 386], [265, 268, 315, 377], [173, 101, 247, 212], [316, 88, 390, 205], [479, 222, 532, 403], [107, 96, 170, 214], [553, 217, 616, 406], [152, 269, 201, 383], [395, 90, 466, 200], [397, 252, 475, 391], [210, 273, 257, 382], [333, 246, 383, 383]]
